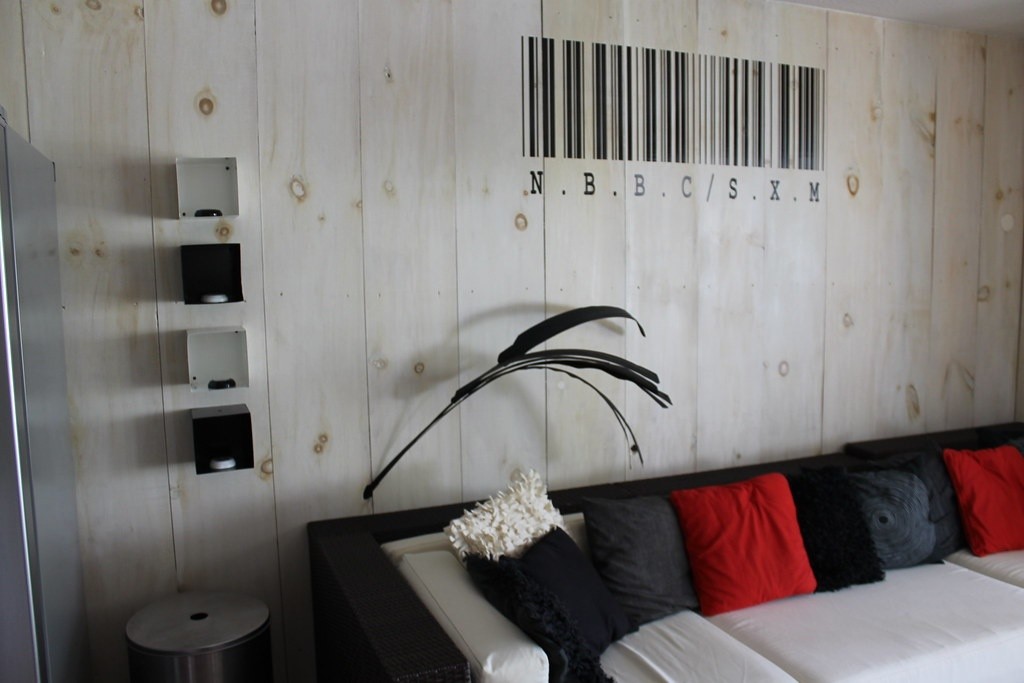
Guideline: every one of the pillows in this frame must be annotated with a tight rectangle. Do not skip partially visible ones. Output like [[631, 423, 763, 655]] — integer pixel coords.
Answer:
[[675, 478, 816, 617], [578, 496, 700, 633], [943, 445, 1024, 557], [790, 478, 885, 595], [381, 513, 585, 565], [400, 551, 549, 683], [839, 456, 952, 568], [449, 467, 567, 567], [464, 528, 637, 683]]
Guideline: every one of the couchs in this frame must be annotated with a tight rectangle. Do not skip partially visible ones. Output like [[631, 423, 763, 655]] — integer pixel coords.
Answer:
[[306, 419, 1024, 683]]
